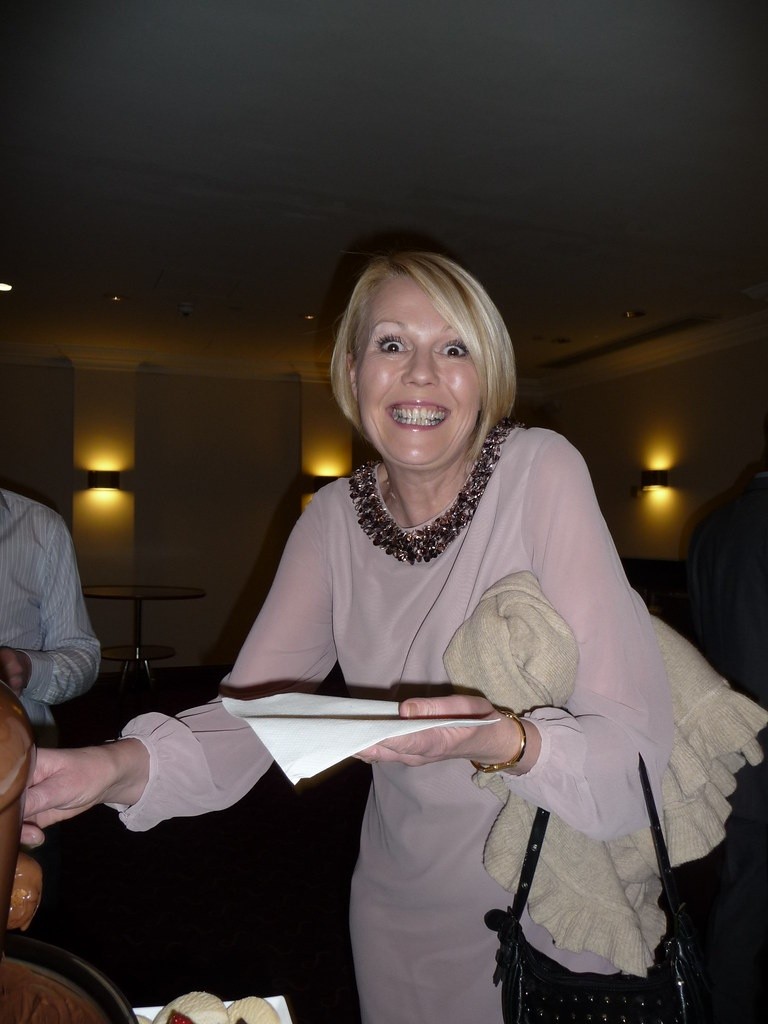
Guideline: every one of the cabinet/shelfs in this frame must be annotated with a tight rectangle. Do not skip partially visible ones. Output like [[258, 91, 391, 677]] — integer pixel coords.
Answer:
[[84, 585, 207, 704]]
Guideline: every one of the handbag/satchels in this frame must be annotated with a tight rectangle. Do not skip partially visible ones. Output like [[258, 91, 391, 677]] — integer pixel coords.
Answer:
[[484, 753, 715, 1024]]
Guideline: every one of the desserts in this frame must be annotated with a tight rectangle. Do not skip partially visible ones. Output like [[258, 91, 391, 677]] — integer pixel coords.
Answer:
[[135, 992, 281, 1024]]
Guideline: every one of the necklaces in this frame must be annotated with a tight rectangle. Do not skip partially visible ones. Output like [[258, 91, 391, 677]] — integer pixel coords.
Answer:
[[349, 417, 528, 566]]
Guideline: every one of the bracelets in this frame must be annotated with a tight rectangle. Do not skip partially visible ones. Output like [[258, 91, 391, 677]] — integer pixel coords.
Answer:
[[18, 249, 676, 1024], [469, 709, 528, 774]]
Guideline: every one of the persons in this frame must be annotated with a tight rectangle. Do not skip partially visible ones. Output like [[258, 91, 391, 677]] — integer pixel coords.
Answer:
[[0, 485, 102, 965]]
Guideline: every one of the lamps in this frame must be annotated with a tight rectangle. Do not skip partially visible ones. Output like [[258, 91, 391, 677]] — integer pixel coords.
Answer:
[[639, 469, 670, 492], [86, 470, 121, 492], [314, 476, 337, 493]]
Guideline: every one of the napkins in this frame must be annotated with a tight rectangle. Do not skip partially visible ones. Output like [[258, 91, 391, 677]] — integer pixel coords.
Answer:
[[222, 692, 502, 785]]
